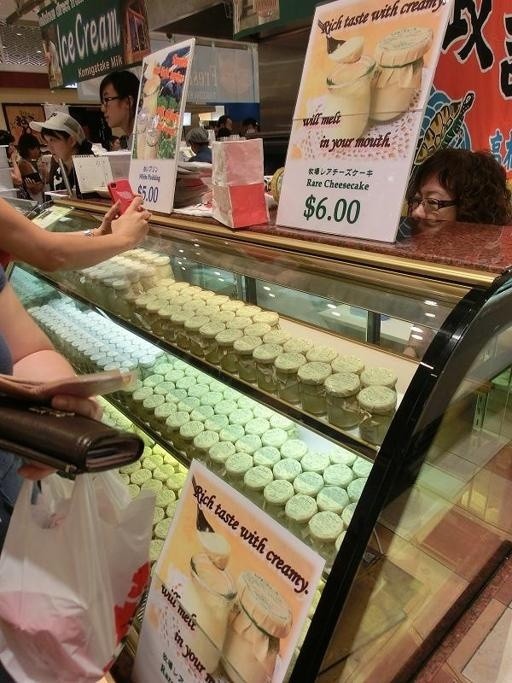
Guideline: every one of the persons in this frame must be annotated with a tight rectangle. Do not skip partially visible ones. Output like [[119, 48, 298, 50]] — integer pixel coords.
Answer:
[[0, 129, 23, 186], [0, 193, 151, 273], [28, 111, 92, 191], [0, 264, 106, 559], [18, 132, 50, 203], [186, 115, 260, 164], [99, 70, 140, 152], [108, 137, 120, 151], [403, 148, 511, 359]]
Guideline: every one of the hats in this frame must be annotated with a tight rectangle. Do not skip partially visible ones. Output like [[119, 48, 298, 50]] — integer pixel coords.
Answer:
[[28, 110, 88, 147], [186, 127, 209, 146]]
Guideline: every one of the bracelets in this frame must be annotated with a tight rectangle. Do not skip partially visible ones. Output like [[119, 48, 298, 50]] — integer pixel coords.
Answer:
[[84, 228, 91, 236], [407, 344, 420, 359]]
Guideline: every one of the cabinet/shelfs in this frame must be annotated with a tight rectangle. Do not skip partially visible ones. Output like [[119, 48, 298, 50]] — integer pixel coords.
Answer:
[[1, 189, 512, 682]]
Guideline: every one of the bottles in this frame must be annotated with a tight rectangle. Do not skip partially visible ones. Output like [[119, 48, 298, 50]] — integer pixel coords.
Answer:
[[155, 555, 291, 682], [41, 161, 49, 180], [319, 27, 434, 145], [137, 73, 161, 160], [8, 229, 401, 668]]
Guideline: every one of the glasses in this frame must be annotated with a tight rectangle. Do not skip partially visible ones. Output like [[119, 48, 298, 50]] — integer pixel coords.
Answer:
[[408, 195, 461, 211], [101, 89, 130, 106], [0, 131, 10, 143]]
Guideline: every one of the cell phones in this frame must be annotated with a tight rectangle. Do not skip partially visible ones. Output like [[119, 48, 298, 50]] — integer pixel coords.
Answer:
[[108, 178, 137, 218]]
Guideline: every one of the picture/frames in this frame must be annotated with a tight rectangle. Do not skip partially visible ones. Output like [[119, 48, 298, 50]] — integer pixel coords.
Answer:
[[1, 102, 48, 149]]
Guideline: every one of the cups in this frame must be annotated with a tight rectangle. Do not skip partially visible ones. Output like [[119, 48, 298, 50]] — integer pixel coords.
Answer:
[[207, 129, 216, 146]]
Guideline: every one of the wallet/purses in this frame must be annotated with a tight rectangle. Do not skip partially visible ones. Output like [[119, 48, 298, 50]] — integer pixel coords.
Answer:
[[0, 403, 146, 479]]
